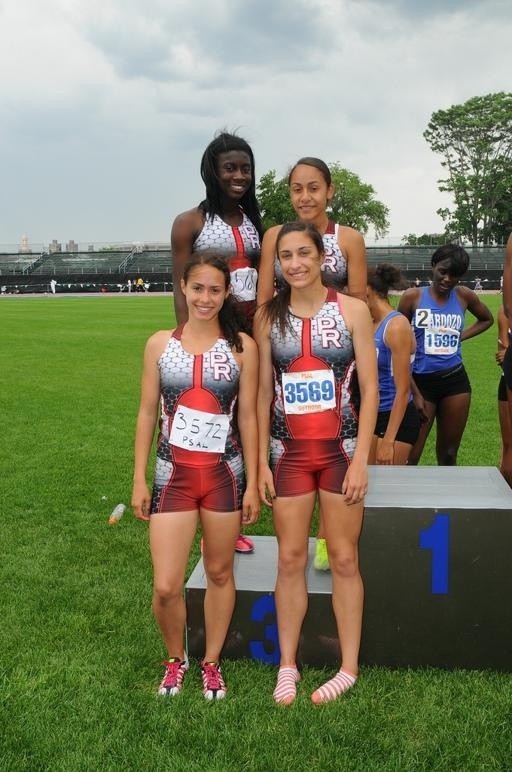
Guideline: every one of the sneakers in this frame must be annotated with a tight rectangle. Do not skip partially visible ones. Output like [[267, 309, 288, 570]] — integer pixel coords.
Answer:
[[312, 538, 330, 571], [201, 661, 228, 702], [235, 533, 254, 552], [158, 650, 189, 698]]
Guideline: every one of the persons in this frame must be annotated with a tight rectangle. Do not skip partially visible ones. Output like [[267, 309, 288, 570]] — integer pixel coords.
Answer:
[[496, 304, 511, 485], [129, 250, 260, 701], [170, 124, 267, 553], [126, 277, 151, 293], [396, 243, 494, 465], [498, 276, 503, 295], [250, 221, 381, 708], [474, 276, 483, 294], [50, 279, 57, 294], [257, 156, 369, 571], [343, 262, 421, 465], [502, 232, 512, 424]]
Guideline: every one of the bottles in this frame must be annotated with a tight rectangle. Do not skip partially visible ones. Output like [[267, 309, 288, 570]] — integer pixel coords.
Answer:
[[107, 503, 127, 526]]
